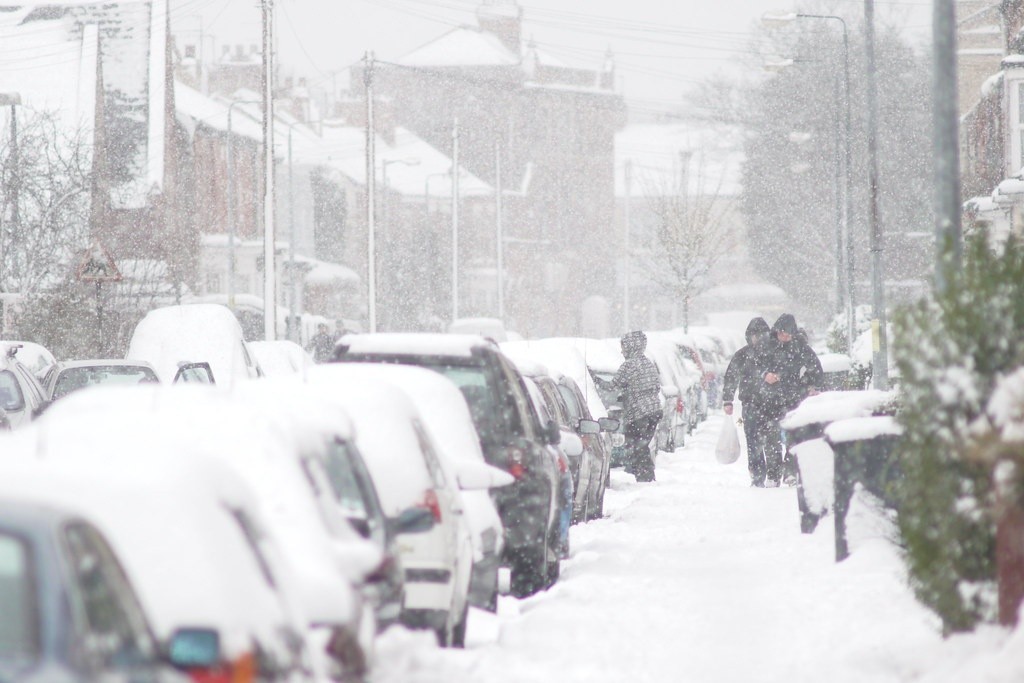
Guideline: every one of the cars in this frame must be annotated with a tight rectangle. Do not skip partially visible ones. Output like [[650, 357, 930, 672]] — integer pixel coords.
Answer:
[[0, 304, 517, 681], [333, 331, 734, 600]]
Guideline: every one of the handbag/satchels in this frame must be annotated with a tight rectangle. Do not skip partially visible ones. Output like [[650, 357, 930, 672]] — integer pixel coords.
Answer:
[[715, 414, 740, 464]]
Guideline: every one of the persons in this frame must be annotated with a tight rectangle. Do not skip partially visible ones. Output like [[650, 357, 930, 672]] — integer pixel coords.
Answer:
[[797, 326, 809, 341], [587, 331, 663, 482], [723, 316, 783, 488], [333, 320, 355, 341], [755, 313, 823, 485], [304, 323, 332, 363]]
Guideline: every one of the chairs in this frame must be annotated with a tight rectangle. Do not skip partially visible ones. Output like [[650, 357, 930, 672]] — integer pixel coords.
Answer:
[[460, 385, 496, 426]]
